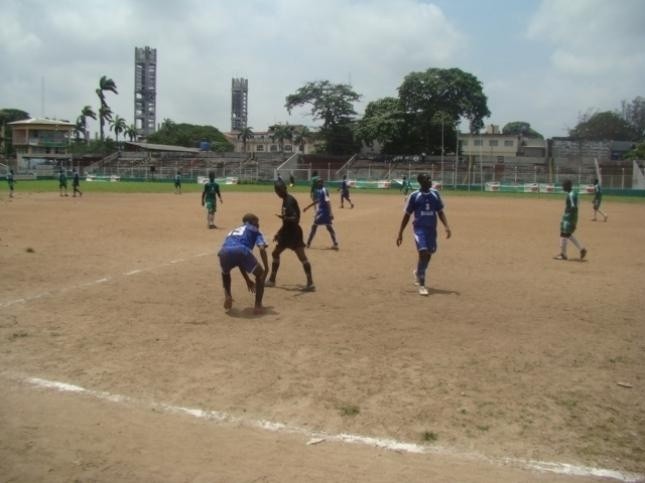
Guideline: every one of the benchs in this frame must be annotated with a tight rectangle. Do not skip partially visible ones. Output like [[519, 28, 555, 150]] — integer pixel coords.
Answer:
[[335, 153, 600, 188], [90, 149, 290, 187]]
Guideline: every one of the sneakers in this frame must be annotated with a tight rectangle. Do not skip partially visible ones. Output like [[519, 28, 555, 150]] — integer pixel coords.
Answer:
[[412, 268, 430, 296], [552, 252, 568, 260], [300, 284, 316, 292], [330, 245, 339, 249], [254, 306, 265, 314], [580, 247, 587, 259], [59, 192, 82, 198], [263, 279, 276, 287], [223, 297, 232, 309], [340, 204, 354, 208], [207, 224, 215, 229], [592, 216, 608, 223], [304, 242, 309, 248]]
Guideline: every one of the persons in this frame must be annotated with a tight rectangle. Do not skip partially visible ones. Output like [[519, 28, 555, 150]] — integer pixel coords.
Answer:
[[587, 178, 610, 222], [552, 179, 587, 261], [71, 169, 83, 198], [173, 172, 187, 196], [6, 169, 18, 200], [398, 176, 411, 200], [393, 172, 453, 297], [57, 169, 69, 198], [199, 167, 355, 310]]
